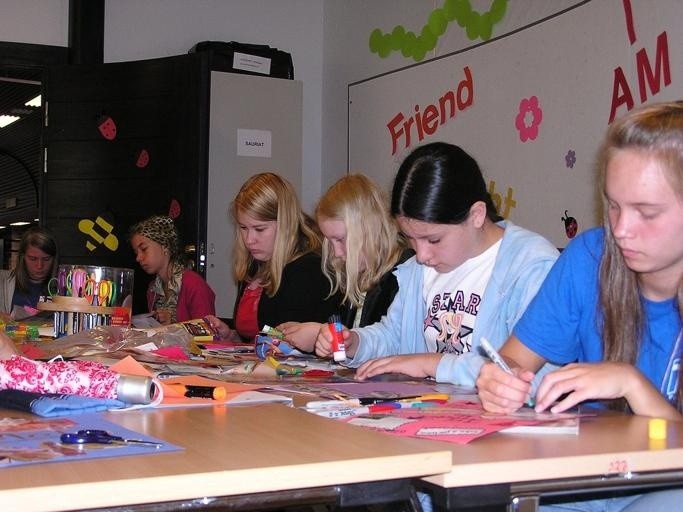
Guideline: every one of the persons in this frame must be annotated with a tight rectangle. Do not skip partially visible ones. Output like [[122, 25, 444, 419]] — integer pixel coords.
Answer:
[[0, 329, 19, 362], [267, 172, 416, 356], [314, 141, 562, 401], [475, 100, 682, 511], [127, 214, 216, 327], [204, 173, 345, 341], [1, 225, 58, 321]]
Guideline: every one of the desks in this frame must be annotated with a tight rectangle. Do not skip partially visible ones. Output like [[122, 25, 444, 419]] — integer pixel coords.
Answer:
[[0, 337, 683, 512]]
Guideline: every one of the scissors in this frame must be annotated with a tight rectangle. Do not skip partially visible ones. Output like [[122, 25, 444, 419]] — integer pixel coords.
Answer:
[[60, 429, 163, 447], [284, 369, 335, 377], [47, 268, 117, 339]]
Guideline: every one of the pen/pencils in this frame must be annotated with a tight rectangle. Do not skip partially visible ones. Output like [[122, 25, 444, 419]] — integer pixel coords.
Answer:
[[306, 393, 450, 416], [159, 382, 226, 401], [479, 337, 534, 407]]
